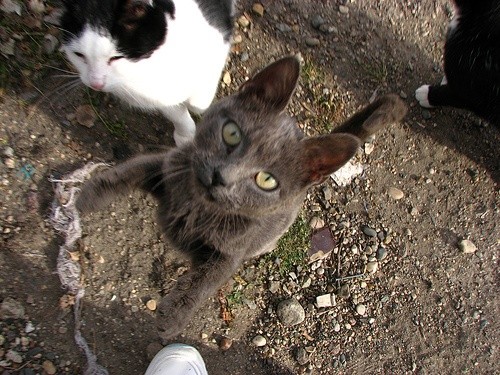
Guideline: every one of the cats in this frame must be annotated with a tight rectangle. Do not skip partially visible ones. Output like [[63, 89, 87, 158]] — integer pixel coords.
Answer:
[[40, 0, 235, 149], [415, 0, 500, 126], [75, 56, 408, 339]]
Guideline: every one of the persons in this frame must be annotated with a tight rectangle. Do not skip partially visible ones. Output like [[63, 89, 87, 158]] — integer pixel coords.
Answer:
[[144, 343, 209, 375]]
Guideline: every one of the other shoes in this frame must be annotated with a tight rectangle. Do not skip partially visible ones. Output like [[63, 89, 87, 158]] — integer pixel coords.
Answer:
[[145, 342, 209, 375]]
